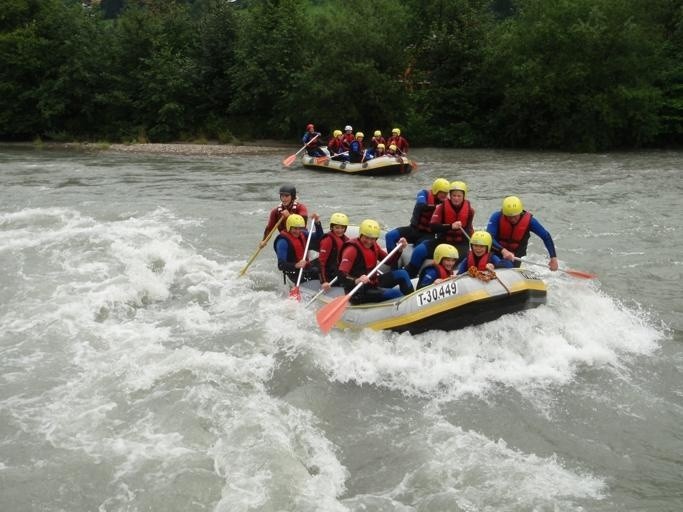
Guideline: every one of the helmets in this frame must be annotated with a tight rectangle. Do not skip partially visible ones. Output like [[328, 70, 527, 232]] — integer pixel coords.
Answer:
[[377, 143, 385, 152], [330, 213, 349, 233], [502, 196, 523, 216], [286, 214, 306, 232], [333, 130, 342, 138], [306, 124, 314, 131], [432, 178, 450, 195], [449, 181, 467, 197], [433, 243, 459, 264], [355, 132, 365, 139], [469, 231, 492, 253], [392, 128, 400, 136], [278, 184, 296, 196], [374, 130, 381, 136], [359, 219, 380, 240], [389, 144, 396, 151], [344, 125, 352, 131]]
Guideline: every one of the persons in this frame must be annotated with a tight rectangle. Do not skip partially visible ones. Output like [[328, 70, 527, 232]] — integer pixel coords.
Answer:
[[430, 181, 475, 267], [486, 196, 558, 273], [385, 178, 450, 278], [258, 184, 308, 248], [454, 231, 512, 282], [302, 124, 408, 162], [416, 243, 460, 290], [318, 212, 349, 295], [337, 219, 414, 305], [273, 214, 324, 285]]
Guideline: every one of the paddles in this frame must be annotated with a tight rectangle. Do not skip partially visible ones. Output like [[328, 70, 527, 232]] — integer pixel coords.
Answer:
[[284, 135, 318, 166], [290, 217, 315, 302], [317, 151, 349, 164], [397, 147, 417, 169], [317, 242, 402, 336], [514, 257, 597, 279]]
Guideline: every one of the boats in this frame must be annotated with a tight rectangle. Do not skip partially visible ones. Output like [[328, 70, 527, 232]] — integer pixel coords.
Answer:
[[303, 154, 412, 176], [288, 267, 547, 337]]
